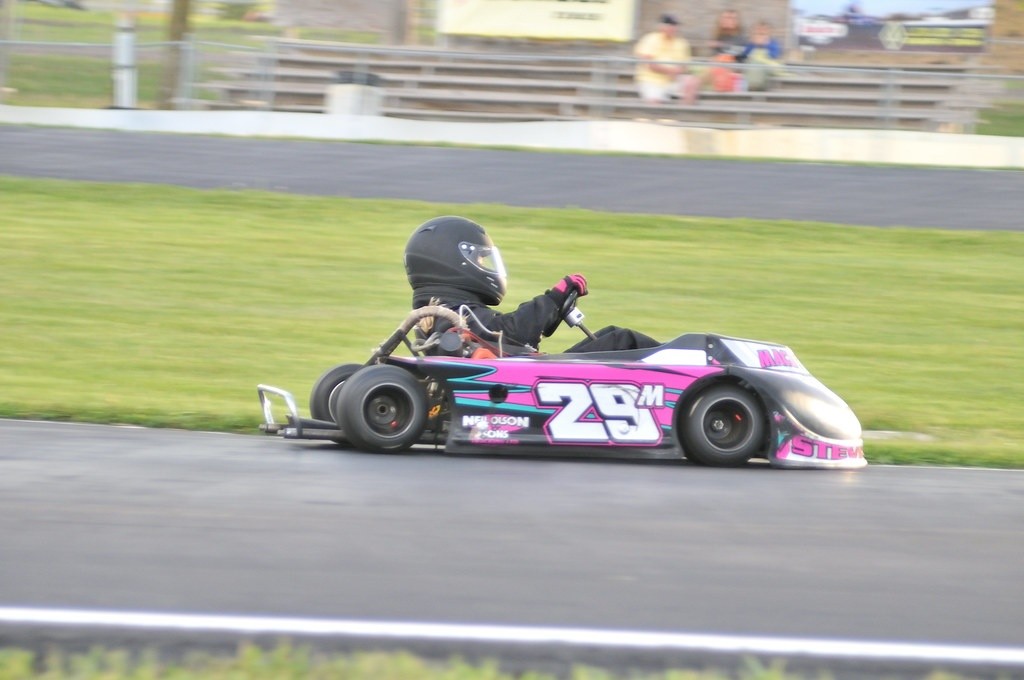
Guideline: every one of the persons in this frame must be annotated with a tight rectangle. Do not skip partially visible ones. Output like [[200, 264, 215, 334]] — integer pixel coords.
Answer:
[[634, 9, 782, 105], [404, 216, 663, 363]]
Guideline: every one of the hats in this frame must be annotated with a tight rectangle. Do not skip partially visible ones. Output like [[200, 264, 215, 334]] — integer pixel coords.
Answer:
[[658, 14, 680, 25]]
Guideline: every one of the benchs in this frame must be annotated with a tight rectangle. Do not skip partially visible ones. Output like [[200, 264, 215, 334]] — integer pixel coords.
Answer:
[[179, 36, 976, 130]]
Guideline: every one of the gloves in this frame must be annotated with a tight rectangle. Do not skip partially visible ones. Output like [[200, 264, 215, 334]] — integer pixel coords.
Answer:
[[545, 274, 588, 306]]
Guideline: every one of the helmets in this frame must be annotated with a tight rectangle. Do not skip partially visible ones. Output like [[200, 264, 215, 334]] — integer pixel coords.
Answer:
[[404, 216, 505, 310]]
